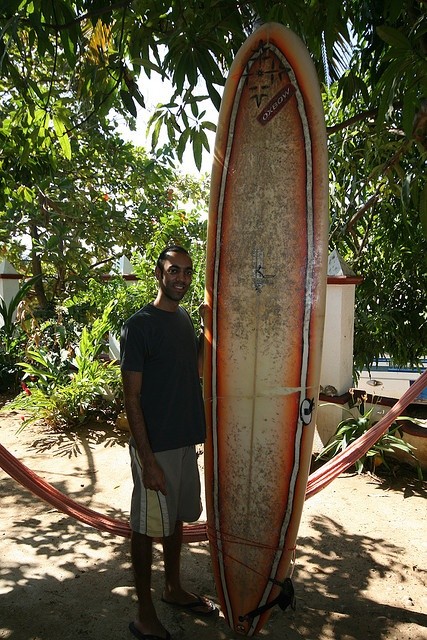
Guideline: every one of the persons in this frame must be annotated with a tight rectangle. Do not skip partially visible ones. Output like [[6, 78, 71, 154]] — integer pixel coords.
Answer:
[[120, 246, 216, 639]]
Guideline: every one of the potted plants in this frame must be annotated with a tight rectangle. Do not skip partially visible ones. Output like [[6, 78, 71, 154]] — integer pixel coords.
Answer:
[[316, 403, 424, 482]]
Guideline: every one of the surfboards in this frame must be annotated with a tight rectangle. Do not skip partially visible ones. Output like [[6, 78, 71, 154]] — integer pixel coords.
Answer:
[[202, 22, 328, 640]]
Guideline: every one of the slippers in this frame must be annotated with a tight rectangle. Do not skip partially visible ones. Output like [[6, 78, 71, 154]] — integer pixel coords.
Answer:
[[160, 592, 215, 616], [128, 622, 170, 640]]
